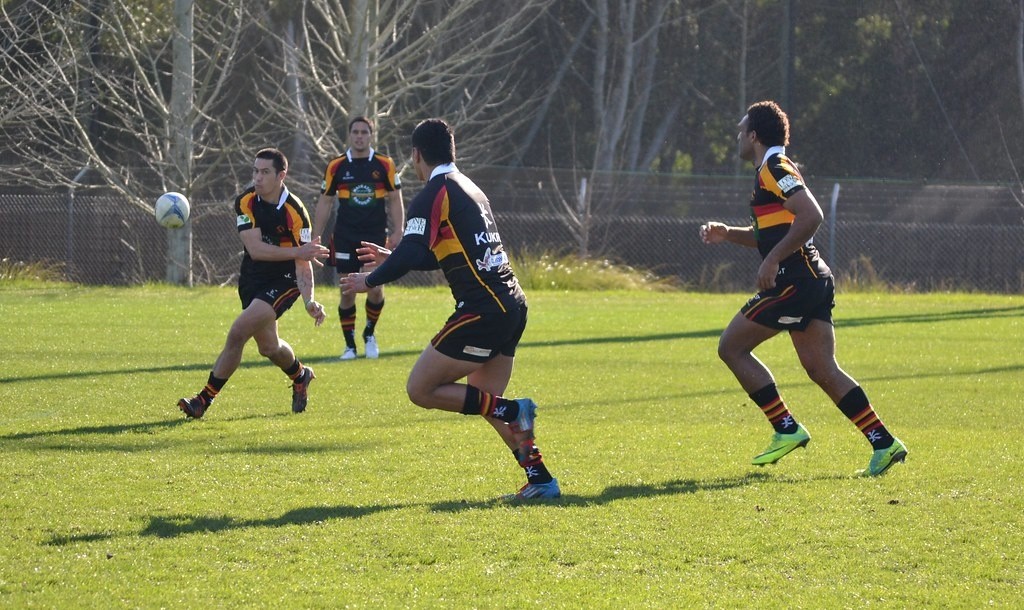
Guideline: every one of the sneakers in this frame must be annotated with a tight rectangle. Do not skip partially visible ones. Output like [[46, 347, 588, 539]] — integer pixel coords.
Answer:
[[504, 398, 537, 462], [498, 478, 560, 500], [340, 346, 357, 359], [363, 332, 378, 359], [751, 423, 811, 466], [177, 394, 211, 419], [288, 365, 316, 413], [862, 437, 908, 477]]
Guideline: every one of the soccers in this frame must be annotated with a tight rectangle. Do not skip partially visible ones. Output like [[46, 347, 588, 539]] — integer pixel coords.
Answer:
[[154, 191, 191, 229]]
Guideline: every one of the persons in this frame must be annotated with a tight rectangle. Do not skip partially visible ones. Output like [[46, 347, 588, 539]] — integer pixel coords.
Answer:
[[178, 148, 330, 420], [315, 117, 405, 362], [698, 101, 908, 479], [338, 117, 560, 501]]
